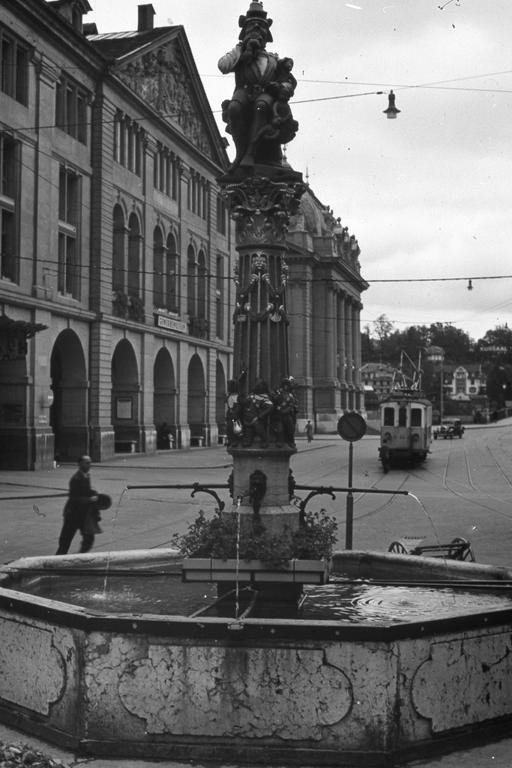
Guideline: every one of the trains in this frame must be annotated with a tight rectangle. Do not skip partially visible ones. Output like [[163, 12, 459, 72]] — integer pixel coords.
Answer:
[[377, 387, 434, 475]]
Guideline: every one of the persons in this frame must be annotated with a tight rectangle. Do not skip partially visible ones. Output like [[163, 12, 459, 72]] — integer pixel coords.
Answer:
[[269, 375, 296, 448], [55, 455, 99, 555], [305, 421, 312, 442], [218, 0, 298, 172], [237, 369, 273, 448]]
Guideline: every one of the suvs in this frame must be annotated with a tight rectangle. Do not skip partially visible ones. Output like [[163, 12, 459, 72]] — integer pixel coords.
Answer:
[[433, 417, 464, 439]]
